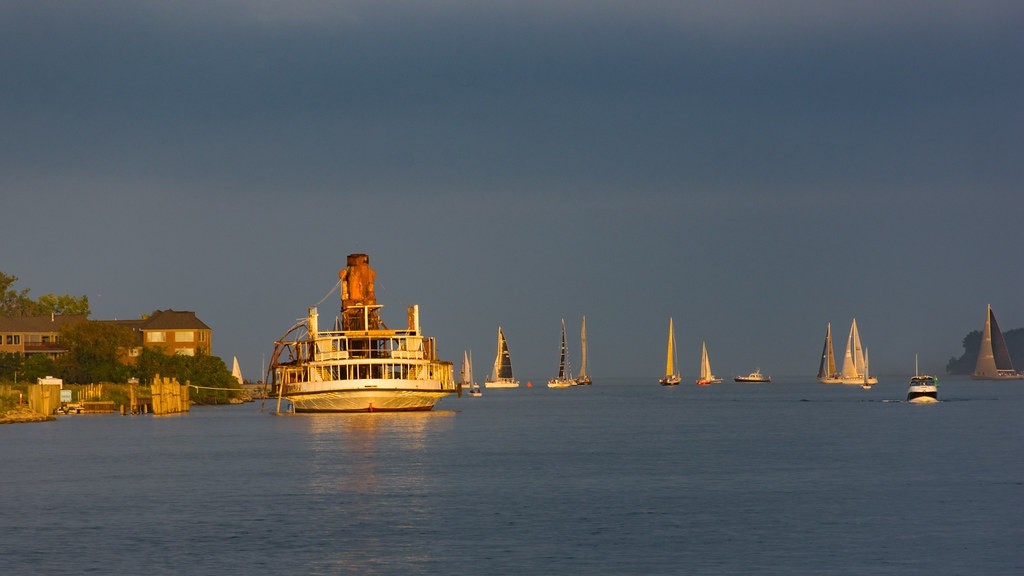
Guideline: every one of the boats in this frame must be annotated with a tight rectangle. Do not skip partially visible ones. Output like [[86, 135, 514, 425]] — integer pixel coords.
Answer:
[[907, 354, 941, 404], [733, 369, 772, 384], [468, 382, 483, 397], [266, 253, 459, 414]]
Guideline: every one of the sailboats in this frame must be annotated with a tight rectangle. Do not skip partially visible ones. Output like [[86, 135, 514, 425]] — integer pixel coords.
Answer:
[[971, 303, 1024, 381], [815, 318, 879, 391], [547, 318, 577, 389], [696, 341, 724, 387], [484, 326, 522, 389], [457, 349, 475, 390], [574, 315, 596, 386], [658, 317, 683, 385]]
